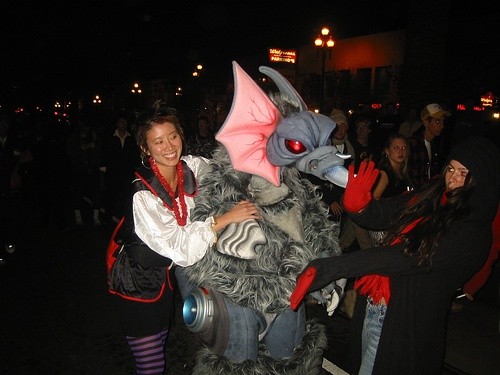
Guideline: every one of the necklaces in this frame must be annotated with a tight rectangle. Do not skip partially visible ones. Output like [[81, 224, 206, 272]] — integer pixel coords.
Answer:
[[148, 157, 188, 226]]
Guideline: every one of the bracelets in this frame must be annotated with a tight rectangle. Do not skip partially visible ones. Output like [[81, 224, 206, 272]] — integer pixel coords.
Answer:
[[211, 216, 224, 233]]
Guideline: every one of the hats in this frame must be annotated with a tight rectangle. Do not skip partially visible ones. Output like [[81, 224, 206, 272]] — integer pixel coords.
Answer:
[[329, 108, 348, 131], [447, 137, 500, 203], [421, 104, 451, 121]]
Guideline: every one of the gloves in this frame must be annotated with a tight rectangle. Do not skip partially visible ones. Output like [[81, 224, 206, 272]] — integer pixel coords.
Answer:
[[290, 258, 319, 312], [343, 160, 380, 212], [351, 272, 392, 306]]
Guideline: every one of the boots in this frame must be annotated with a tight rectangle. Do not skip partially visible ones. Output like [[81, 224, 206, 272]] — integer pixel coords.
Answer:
[[75, 209, 82, 224], [93, 208, 101, 225]]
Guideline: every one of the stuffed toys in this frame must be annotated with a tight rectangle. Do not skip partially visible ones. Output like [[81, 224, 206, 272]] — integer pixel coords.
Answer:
[[178, 97, 344, 375]]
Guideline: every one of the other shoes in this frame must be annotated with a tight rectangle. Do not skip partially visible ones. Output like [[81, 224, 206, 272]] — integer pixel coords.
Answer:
[[99, 208, 106, 221], [0, 245, 9, 265], [5, 239, 16, 253]]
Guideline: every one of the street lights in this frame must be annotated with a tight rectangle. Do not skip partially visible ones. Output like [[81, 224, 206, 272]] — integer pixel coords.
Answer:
[[314, 26, 335, 109], [131, 82, 143, 112]]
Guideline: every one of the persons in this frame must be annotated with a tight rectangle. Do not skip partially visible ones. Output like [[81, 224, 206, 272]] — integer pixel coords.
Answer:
[[107, 110, 260, 375], [0, 91, 227, 226], [289, 102, 500, 375]]
